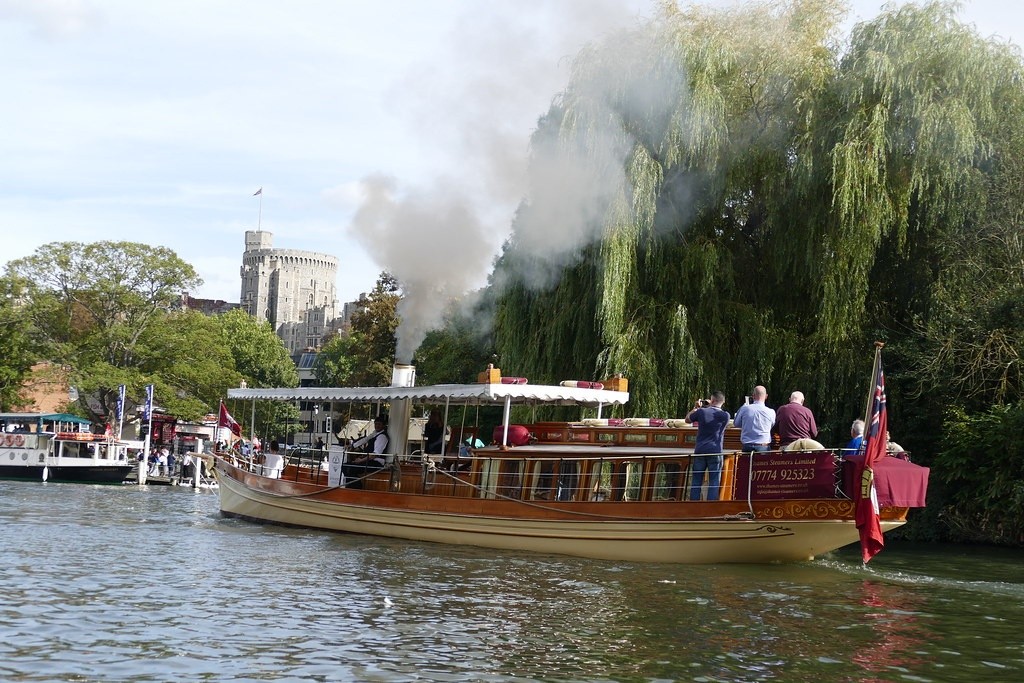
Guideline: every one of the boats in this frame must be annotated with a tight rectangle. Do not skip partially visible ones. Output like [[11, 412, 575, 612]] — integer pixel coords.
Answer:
[[207, 355, 932, 567], [0, 412, 135, 485]]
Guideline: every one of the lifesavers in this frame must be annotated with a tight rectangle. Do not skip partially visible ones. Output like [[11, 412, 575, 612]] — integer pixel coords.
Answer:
[[784, 438, 825, 450], [560, 380, 604, 390], [623, 418, 664, 427], [666, 418, 699, 427], [5, 435, 14, 445], [15, 434, 25, 446], [501, 376, 529, 385], [581, 418, 622, 427], [0, 433, 5, 445]]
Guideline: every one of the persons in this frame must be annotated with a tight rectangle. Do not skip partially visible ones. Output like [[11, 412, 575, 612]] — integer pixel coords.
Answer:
[[341, 416, 390, 489], [66, 444, 108, 459], [733, 386, 776, 454], [11, 423, 79, 434], [136, 433, 323, 479], [843, 419, 868, 459], [774, 391, 817, 450], [685, 390, 730, 500]]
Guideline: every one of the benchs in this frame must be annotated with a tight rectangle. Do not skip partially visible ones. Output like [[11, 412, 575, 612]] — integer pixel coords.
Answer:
[[281, 463, 472, 497]]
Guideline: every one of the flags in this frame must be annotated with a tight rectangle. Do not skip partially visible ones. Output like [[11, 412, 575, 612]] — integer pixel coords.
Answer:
[[218, 403, 241, 438], [853, 346, 887, 562]]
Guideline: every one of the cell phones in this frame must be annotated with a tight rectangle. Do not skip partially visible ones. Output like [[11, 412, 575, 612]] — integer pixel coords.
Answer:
[[745, 396, 750, 405], [701, 401, 708, 405]]
